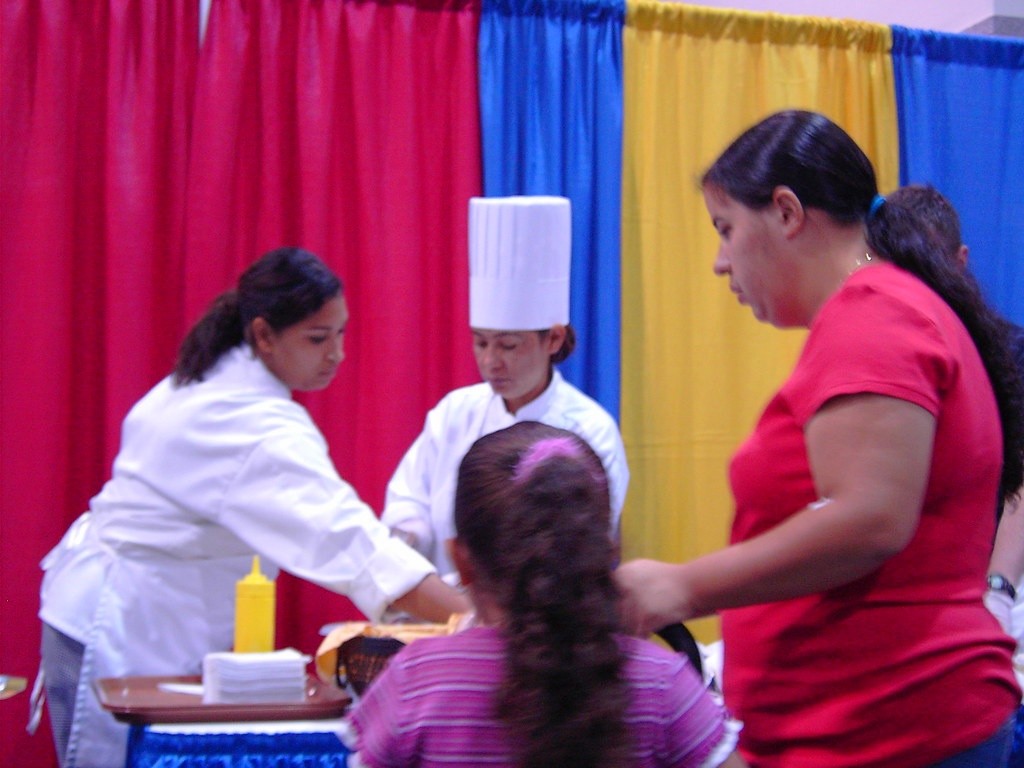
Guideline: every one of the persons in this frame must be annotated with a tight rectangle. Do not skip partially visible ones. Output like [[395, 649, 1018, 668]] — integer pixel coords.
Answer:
[[379, 194, 631, 596], [35, 243, 474, 767], [347, 418, 746, 768], [616, 109, 1024, 768]]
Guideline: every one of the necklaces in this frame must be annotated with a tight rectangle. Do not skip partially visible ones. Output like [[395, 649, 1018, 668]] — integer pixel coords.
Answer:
[[838, 252, 872, 282]]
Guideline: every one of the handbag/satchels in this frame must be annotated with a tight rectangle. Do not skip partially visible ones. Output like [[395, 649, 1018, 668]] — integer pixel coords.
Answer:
[[335, 637, 405, 698]]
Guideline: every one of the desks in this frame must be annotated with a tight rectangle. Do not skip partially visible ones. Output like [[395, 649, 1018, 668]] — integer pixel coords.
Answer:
[[90, 676, 352, 768]]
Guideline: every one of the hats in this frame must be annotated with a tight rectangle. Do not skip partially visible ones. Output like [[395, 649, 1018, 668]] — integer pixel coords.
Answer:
[[468, 196, 570, 332]]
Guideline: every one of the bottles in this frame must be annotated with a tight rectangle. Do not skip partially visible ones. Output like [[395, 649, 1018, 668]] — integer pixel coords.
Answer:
[[234, 555, 276, 654]]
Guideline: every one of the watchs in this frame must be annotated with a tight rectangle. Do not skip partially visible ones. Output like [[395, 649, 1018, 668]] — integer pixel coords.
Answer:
[[987, 573, 1015, 598]]
